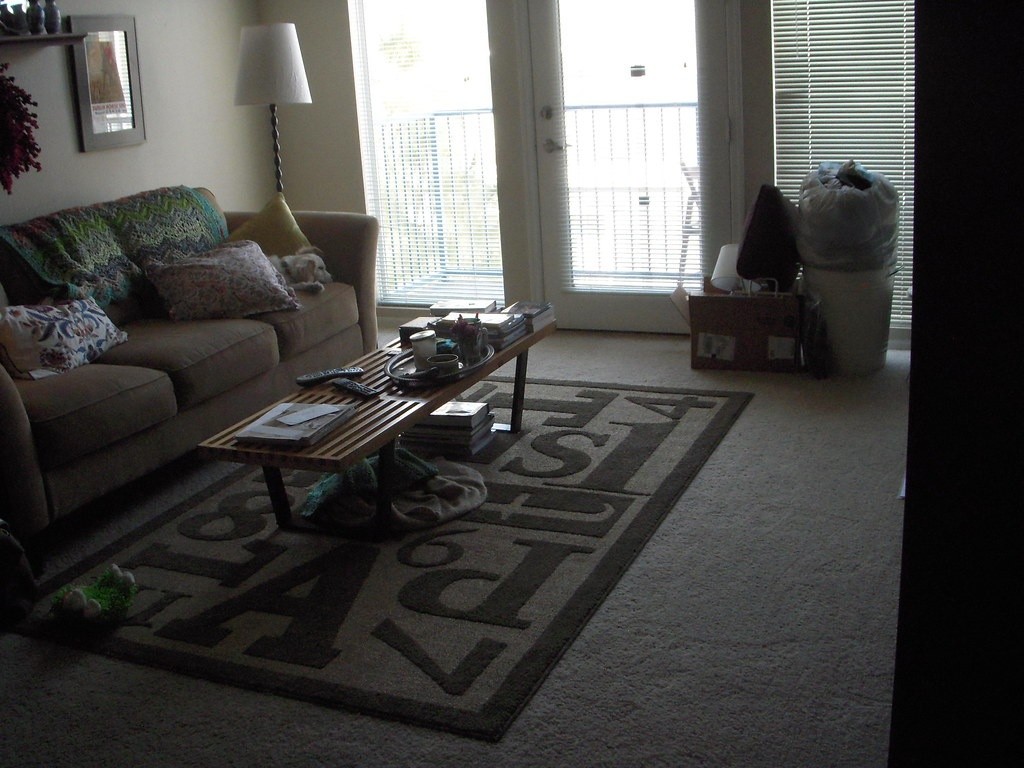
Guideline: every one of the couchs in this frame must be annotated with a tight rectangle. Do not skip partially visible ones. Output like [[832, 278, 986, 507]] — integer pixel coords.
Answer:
[[0, 185, 379, 542]]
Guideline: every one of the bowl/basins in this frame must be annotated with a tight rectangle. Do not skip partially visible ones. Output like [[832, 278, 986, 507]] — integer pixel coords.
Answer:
[[426, 354, 459, 375]]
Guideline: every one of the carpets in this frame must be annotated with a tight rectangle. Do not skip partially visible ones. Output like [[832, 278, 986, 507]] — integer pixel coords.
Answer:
[[1, 374, 756, 742]]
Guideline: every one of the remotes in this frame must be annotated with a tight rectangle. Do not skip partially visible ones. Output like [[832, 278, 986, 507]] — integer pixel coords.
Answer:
[[296, 367, 363, 387], [333, 377, 378, 397]]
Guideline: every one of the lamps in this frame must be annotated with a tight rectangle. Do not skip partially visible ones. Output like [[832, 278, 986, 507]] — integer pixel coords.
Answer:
[[710, 243, 763, 295], [232, 23, 312, 190]]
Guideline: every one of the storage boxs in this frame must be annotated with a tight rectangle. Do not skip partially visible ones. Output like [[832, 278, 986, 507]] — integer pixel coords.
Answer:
[[670, 278, 802, 373]]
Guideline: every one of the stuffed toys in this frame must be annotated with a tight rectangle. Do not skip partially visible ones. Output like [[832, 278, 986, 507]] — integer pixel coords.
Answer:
[[51, 563, 137, 625]]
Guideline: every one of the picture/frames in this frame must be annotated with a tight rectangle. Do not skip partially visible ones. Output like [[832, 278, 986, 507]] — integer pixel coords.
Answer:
[[67, 14, 150, 153]]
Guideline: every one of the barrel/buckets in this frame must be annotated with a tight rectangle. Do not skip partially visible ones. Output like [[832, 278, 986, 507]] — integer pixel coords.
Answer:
[[795, 261, 906, 375]]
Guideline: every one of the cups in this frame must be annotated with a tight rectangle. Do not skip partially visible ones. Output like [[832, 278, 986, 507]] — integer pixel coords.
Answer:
[[455, 319, 489, 362]]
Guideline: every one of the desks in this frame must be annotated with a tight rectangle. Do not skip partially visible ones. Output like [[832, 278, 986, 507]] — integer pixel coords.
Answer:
[[198, 318, 558, 528]]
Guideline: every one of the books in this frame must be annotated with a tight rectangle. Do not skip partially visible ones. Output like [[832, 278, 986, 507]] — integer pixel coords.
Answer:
[[398, 300, 555, 354], [398, 402, 496, 456], [235, 401, 358, 446]]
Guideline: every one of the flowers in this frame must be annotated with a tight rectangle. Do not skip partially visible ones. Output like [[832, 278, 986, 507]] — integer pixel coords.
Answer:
[[0, 62, 42, 194]]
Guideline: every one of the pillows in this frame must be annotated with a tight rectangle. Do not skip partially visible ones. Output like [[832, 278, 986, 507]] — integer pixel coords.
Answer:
[[147, 192, 314, 319], [736, 185, 801, 292], [1, 293, 127, 382]]
